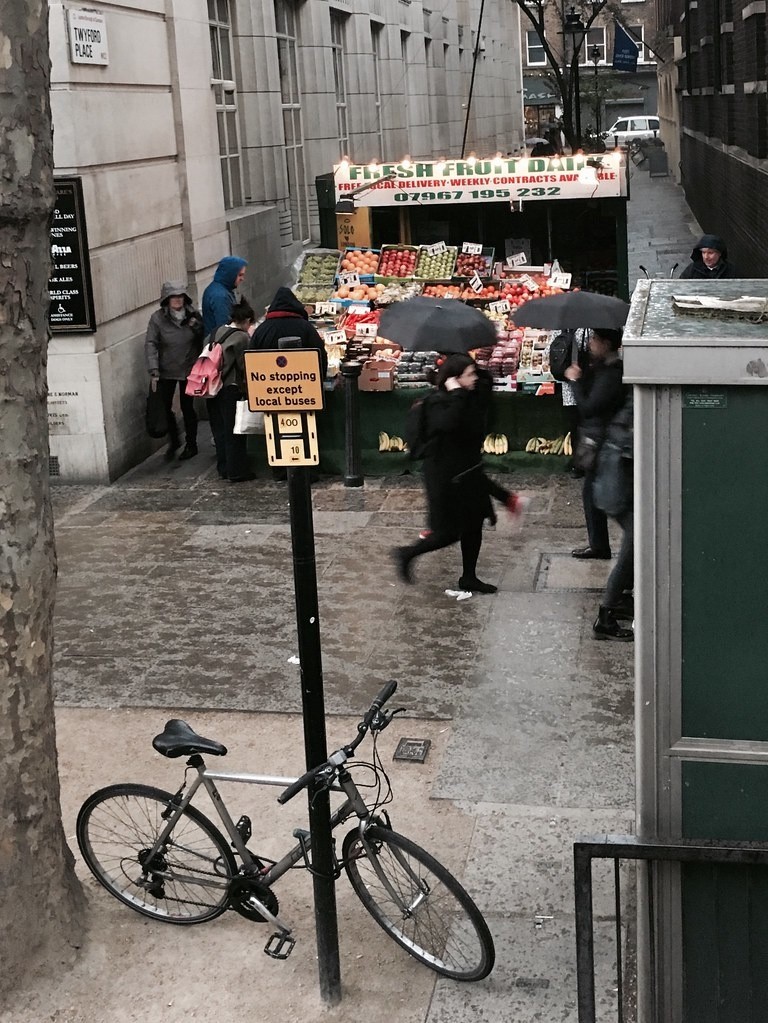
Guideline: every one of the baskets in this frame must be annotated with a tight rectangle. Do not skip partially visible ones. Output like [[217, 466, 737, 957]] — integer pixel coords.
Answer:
[[293, 244, 503, 313]]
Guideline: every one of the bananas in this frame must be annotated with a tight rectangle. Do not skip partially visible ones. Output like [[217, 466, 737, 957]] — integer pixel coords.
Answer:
[[378, 431, 408, 451], [526, 431, 572, 455], [480, 432, 509, 454]]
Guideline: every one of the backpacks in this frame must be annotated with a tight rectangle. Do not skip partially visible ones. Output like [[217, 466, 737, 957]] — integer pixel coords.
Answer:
[[549, 330, 575, 382], [184, 328, 248, 397]]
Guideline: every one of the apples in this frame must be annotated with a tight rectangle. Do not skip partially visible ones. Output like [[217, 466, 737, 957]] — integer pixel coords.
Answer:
[[332, 283, 386, 300], [455, 253, 487, 276], [379, 249, 416, 277], [416, 250, 454, 279], [341, 250, 379, 275], [499, 272, 561, 311], [461, 284, 499, 299], [375, 336, 402, 358], [423, 285, 460, 300]]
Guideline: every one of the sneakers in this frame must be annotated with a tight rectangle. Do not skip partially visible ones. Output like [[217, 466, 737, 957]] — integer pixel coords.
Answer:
[[164, 437, 182, 462], [178, 444, 198, 461]]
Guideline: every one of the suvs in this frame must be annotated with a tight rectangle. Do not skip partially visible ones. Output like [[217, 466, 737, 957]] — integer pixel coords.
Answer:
[[587, 114, 662, 154]]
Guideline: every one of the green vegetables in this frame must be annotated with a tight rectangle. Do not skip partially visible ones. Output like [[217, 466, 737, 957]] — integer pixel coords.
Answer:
[[293, 255, 339, 302]]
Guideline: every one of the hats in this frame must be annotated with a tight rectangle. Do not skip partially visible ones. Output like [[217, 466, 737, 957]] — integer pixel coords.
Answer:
[[591, 327, 621, 347]]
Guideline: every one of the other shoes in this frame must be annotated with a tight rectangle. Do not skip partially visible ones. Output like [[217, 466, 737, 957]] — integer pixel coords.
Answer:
[[421, 529, 433, 537], [227, 471, 257, 482], [459, 577, 497, 594], [218, 473, 227, 478], [565, 459, 574, 472], [573, 467, 586, 479], [398, 547, 413, 585], [508, 496, 530, 522]]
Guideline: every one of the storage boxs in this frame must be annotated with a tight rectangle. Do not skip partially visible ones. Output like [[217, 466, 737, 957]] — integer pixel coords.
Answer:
[[291, 243, 558, 392]]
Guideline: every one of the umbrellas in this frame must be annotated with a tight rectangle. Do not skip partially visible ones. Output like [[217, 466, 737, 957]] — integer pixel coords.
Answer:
[[509, 291, 629, 331], [523, 138, 550, 145], [537, 121, 560, 128], [377, 296, 497, 352]]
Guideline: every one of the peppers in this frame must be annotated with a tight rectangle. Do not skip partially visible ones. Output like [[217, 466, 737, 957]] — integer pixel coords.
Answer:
[[344, 310, 382, 330]]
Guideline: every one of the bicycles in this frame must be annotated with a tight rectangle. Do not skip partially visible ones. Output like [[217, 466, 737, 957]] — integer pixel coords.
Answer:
[[74, 678, 496, 988]]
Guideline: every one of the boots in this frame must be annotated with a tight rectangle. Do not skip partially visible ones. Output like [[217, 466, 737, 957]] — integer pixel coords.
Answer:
[[592, 605, 633, 641], [612, 593, 634, 620]]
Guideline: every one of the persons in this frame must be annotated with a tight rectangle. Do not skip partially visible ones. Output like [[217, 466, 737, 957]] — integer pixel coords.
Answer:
[[203, 294, 257, 483], [593, 385, 634, 642], [679, 234, 740, 279], [390, 354, 498, 593], [144, 281, 203, 461], [542, 328, 602, 479], [565, 328, 634, 559], [531, 112, 565, 157], [202, 256, 248, 448], [248, 287, 328, 484], [419, 352, 532, 540]]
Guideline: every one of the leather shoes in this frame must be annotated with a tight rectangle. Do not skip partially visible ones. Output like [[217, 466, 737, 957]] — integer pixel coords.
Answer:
[[572, 547, 611, 559]]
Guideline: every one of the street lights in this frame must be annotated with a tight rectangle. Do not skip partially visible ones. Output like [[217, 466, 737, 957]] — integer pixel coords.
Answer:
[[589, 43, 602, 153], [556, 5, 591, 151]]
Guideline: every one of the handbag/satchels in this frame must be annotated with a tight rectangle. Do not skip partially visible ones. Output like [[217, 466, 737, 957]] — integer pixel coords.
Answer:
[[406, 392, 433, 444], [578, 327, 592, 376], [590, 443, 626, 516], [146, 379, 168, 439], [232, 398, 265, 434], [572, 443, 596, 472]]
